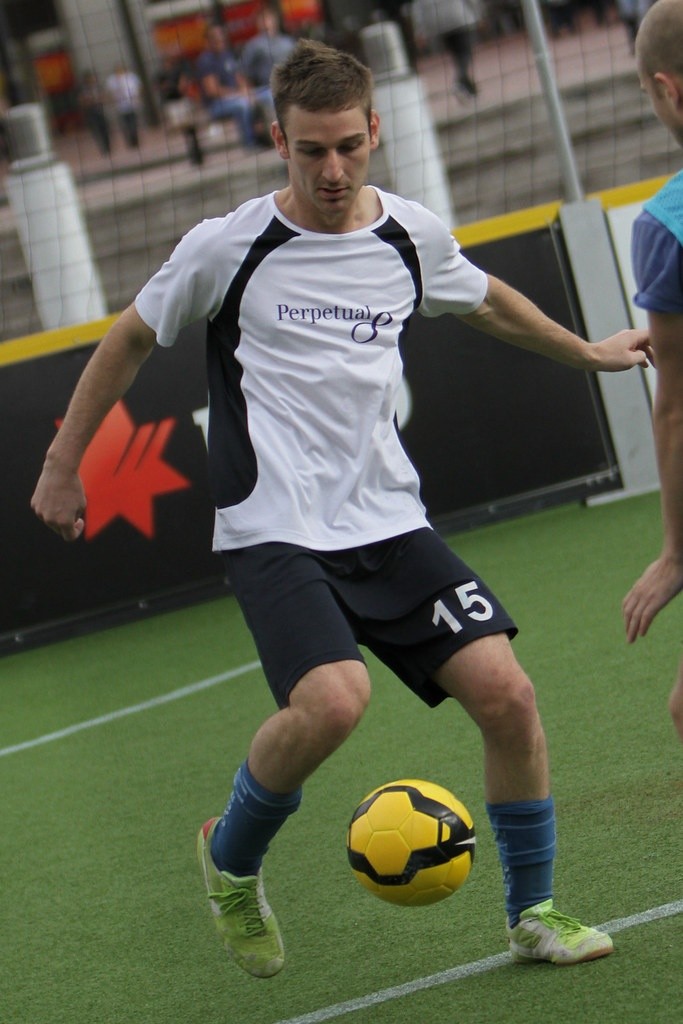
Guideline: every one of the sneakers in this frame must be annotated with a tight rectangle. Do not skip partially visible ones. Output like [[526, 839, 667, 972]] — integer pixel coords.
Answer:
[[506, 899, 614, 965], [197, 817, 285, 979]]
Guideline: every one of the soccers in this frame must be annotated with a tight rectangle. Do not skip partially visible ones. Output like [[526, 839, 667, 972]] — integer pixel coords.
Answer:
[[347, 779, 475, 908]]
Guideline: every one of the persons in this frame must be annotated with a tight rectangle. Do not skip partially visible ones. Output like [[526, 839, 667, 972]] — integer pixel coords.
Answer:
[[31, 39, 657, 979], [622, 0, 683, 744], [67, 0, 658, 170]]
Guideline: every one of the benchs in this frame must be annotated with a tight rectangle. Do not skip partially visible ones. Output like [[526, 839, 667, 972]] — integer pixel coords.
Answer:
[[165, 95, 265, 167]]
